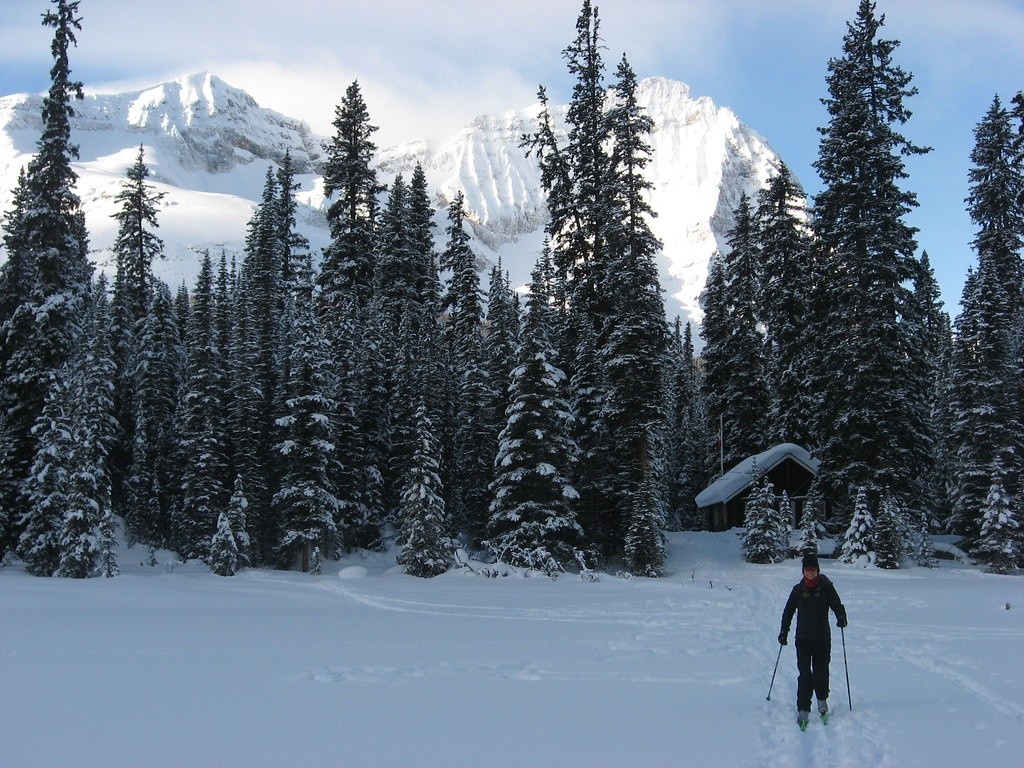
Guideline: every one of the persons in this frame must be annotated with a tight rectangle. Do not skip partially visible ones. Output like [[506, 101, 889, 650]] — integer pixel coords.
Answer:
[[778, 553, 847, 732]]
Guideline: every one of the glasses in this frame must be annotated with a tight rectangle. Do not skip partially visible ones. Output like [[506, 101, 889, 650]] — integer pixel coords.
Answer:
[[804, 568, 816, 572]]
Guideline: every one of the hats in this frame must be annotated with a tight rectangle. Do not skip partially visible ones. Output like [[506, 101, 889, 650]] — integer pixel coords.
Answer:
[[802, 556, 820, 574]]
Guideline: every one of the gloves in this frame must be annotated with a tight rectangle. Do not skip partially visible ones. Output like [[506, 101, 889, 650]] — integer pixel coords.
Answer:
[[778, 633, 787, 645], [837, 618, 847, 628]]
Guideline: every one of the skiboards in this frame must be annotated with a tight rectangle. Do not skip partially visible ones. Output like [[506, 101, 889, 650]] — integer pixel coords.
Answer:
[[795, 701, 829, 732]]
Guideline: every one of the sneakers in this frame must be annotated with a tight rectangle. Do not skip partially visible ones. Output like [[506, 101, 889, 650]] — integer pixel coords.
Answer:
[[816, 698, 828, 712], [796, 711, 809, 723]]
